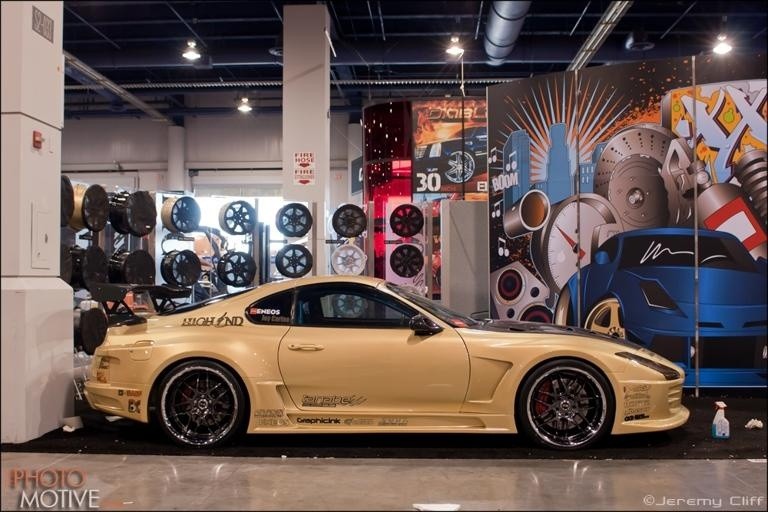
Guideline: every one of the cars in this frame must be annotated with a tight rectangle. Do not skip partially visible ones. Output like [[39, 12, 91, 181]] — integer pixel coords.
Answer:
[[82, 274, 690, 450], [414, 126, 487, 185], [552, 227, 768, 389]]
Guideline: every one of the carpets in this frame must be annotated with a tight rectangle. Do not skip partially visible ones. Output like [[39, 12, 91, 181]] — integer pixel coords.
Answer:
[[0, 388, 767, 459]]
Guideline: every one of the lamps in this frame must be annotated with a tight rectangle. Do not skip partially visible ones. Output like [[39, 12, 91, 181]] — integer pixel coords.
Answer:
[[236, 96, 252, 113], [711, 22, 733, 55], [182, 37, 201, 61], [445, 34, 465, 56]]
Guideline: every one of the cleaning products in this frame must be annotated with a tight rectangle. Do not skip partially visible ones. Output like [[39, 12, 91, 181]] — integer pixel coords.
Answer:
[[713, 401, 730, 439]]
